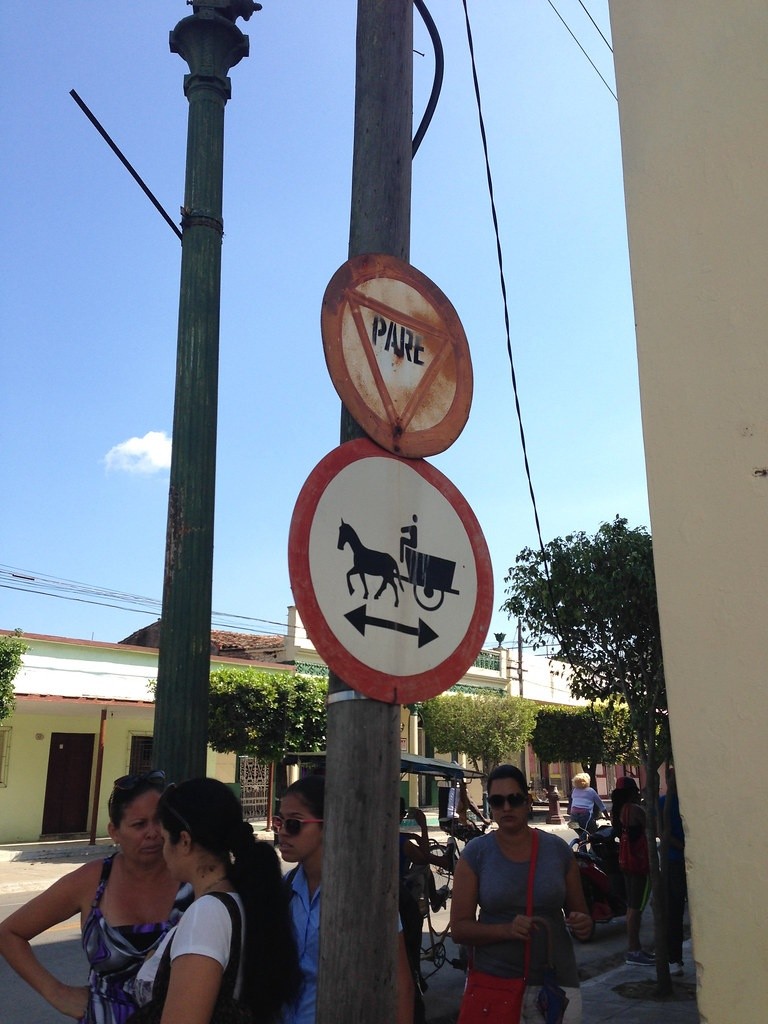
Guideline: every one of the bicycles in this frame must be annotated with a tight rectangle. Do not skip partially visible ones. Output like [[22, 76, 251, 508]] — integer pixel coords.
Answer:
[[569, 816, 610, 854], [429, 820, 493, 894]]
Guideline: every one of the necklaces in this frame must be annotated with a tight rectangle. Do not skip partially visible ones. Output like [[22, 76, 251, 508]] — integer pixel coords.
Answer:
[[203, 877, 230, 894]]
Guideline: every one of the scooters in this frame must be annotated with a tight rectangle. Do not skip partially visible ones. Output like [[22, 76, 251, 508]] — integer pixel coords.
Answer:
[[569, 821, 629, 944]]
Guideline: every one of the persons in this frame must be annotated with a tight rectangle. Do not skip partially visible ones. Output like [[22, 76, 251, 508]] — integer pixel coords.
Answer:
[[438, 761, 491, 842], [450, 764, 592, 1024], [612, 768, 688, 975], [272, 775, 326, 1023], [569, 772, 611, 853], [132, 777, 304, 1023], [0, 770, 195, 1024], [399, 797, 456, 913]]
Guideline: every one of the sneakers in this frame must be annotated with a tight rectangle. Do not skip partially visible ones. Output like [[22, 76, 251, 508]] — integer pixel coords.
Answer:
[[626, 950, 655, 965], [670, 963, 684, 976], [430, 885, 450, 912], [444, 837, 456, 871]]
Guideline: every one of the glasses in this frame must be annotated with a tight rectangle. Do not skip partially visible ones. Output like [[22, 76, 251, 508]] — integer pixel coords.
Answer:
[[271, 815, 324, 835], [486, 793, 525, 810], [158, 781, 190, 834], [109, 768, 167, 818]]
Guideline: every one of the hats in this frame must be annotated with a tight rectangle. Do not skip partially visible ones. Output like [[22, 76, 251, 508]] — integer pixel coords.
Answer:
[[616, 776, 639, 791]]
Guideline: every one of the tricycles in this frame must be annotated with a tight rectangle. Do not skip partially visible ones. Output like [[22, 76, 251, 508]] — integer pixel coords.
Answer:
[[283, 751, 486, 993]]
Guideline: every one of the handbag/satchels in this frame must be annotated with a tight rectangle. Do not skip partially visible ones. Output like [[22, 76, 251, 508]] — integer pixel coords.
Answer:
[[124, 891, 257, 1023], [456, 971, 528, 1023]]
